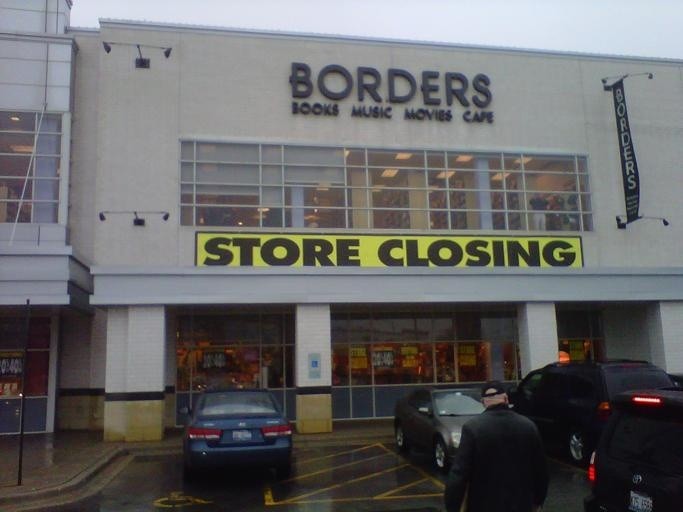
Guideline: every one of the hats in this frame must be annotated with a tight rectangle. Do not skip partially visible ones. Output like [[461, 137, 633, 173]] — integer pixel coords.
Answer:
[[481, 381, 505, 397]]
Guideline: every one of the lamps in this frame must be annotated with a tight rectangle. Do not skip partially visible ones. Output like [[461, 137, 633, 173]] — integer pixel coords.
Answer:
[[99, 210, 169, 226], [102, 41, 172, 68]]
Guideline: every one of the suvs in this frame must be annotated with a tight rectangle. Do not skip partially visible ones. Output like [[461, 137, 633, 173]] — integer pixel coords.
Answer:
[[508, 357, 678, 463], [583, 390, 682, 512]]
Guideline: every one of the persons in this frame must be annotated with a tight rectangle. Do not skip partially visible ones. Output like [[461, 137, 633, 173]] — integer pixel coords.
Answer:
[[529, 192, 551, 230], [442, 379, 550, 511], [565, 183, 579, 231], [544, 195, 560, 230]]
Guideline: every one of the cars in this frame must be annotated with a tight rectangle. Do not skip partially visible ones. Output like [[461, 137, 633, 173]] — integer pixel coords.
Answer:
[[177, 387, 294, 480], [394, 384, 516, 470]]
